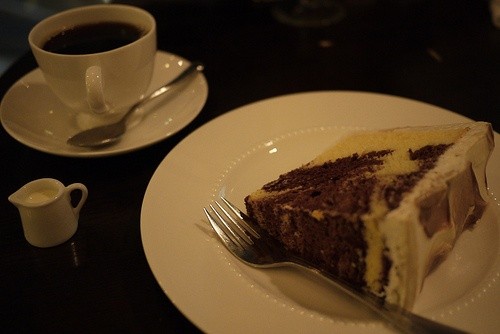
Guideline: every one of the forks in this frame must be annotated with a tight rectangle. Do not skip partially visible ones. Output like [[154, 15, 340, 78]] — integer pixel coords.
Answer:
[[204, 196, 472, 334]]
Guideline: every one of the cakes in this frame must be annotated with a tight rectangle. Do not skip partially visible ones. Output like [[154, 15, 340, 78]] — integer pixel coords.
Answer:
[[244, 121, 494, 312]]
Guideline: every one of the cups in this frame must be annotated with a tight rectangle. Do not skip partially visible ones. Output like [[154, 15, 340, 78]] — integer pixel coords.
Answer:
[[9, 178, 88, 248], [29, 3, 157, 116]]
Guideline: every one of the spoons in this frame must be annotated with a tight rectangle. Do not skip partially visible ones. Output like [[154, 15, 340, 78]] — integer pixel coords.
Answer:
[[66, 63, 202, 146]]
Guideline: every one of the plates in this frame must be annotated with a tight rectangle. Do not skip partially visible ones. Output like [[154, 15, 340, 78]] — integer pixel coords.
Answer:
[[140, 91, 500, 334], [1, 53, 209, 158]]
